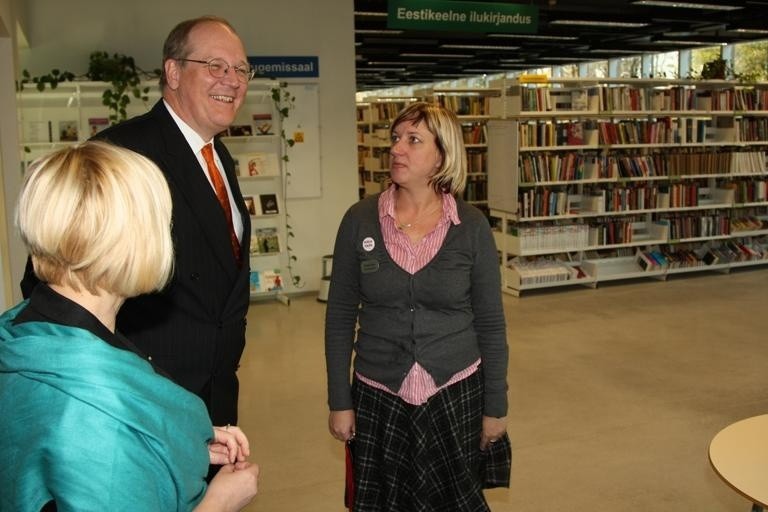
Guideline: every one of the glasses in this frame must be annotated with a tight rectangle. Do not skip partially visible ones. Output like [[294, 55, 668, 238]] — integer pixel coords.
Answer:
[[176, 56, 256, 83]]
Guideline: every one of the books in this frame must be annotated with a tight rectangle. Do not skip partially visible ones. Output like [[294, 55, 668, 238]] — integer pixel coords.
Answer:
[[58, 121, 79, 140], [260, 193, 279, 214], [251, 113, 273, 135], [357, 95, 489, 219], [507, 83, 767, 286], [250, 269, 285, 293], [87, 117, 109, 137], [256, 227, 280, 253]]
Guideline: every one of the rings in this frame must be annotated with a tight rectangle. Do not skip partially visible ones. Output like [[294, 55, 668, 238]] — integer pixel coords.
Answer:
[[490, 439, 497, 443], [226, 424, 231, 429]]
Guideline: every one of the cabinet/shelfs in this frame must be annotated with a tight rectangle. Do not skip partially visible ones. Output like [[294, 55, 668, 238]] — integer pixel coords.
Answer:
[[488, 77, 764, 299], [16, 79, 292, 308], [356, 84, 487, 206]]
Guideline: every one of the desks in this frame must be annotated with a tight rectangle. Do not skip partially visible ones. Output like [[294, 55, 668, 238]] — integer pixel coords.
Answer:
[[709, 413, 768, 512]]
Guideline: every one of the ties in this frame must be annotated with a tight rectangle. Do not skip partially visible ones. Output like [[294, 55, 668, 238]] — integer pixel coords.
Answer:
[[202, 143, 241, 262]]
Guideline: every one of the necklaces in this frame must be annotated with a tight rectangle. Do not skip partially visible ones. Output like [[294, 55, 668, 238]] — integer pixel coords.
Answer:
[[396, 199, 440, 227]]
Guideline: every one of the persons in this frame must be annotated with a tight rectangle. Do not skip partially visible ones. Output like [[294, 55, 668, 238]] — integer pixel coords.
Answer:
[[323, 101, 511, 512], [0, 138, 260, 512], [18, 15, 253, 484]]
